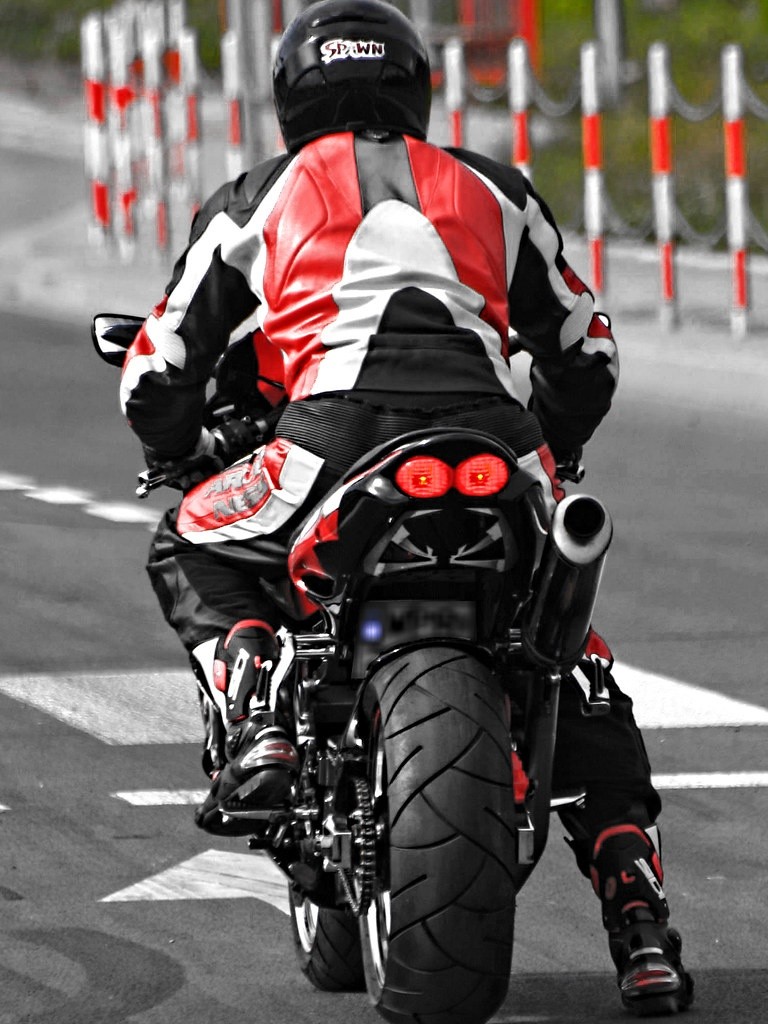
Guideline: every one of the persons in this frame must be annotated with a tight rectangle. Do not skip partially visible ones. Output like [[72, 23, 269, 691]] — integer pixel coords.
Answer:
[[119, 0, 695, 1015]]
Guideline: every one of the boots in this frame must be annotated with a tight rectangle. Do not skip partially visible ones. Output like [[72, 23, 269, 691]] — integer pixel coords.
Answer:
[[193, 620, 299, 835], [562, 820, 695, 1017]]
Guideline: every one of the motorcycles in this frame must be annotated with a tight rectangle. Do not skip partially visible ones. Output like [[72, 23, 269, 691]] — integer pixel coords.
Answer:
[[89, 310, 620, 1024]]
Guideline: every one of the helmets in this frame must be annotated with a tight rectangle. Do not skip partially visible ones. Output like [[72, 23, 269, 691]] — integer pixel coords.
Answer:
[[272, 0, 432, 154]]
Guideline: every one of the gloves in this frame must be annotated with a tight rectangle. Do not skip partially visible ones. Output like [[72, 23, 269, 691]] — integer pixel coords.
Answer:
[[139, 425, 229, 491], [522, 404, 583, 483]]
